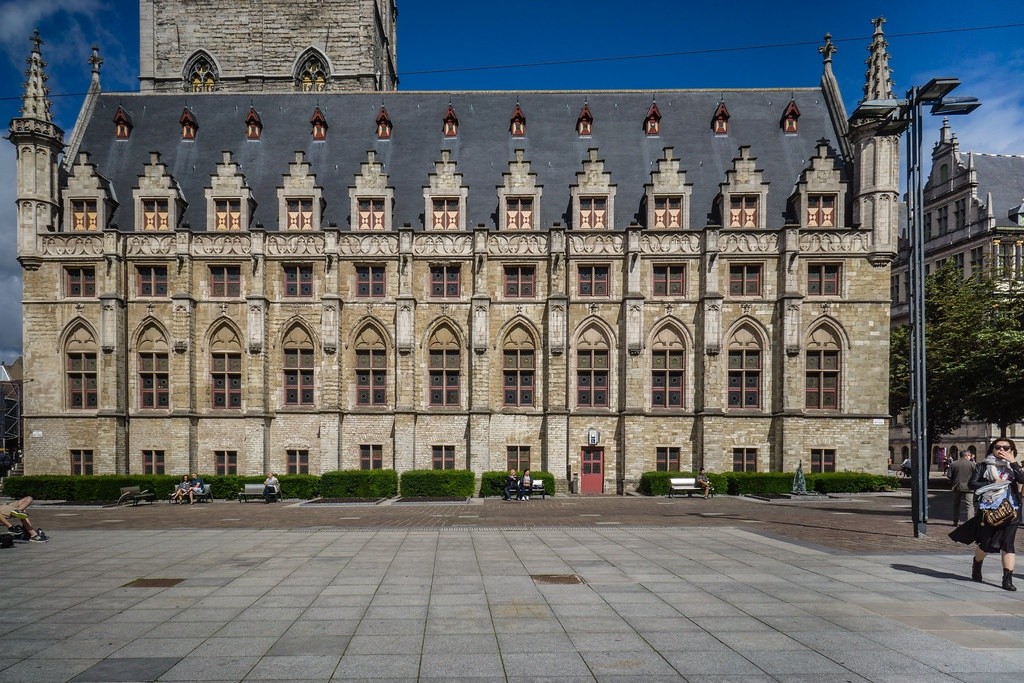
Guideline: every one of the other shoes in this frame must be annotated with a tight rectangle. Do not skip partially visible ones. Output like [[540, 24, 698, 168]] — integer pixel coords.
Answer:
[[517, 498, 520, 501], [521, 496, 525, 501], [507, 498, 510, 501], [526, 496, 529, 500]]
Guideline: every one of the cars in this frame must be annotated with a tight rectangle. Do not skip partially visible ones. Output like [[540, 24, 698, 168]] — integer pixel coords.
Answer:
[[0, 452, 13, 478]]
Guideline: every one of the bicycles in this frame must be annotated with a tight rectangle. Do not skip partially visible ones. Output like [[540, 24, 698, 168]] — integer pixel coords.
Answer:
[[895, 466, 912, 479]]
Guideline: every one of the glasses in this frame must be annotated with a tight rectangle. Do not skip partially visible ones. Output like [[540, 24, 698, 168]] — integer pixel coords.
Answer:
[[993, 445, 1011, 451]]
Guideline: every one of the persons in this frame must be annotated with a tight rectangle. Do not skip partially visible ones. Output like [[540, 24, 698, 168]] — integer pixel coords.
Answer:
[[888, 458, 892, 470], [261, 471, 280, 504], [696, 468, 710, 500], [900, 455, 911, 479], [943, 438, 1024, 591], [172, 472, 205, 505], [519, 469, 533, 501], [501, 469, 520, 501], [0, 496, 48, 543]]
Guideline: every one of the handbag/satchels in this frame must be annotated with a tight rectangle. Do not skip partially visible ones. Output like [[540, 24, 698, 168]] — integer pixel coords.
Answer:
[[981, 498, 1018, 527]]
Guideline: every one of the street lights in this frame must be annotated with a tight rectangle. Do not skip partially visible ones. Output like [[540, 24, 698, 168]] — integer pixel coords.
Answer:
[[847, 75, 985, 536]]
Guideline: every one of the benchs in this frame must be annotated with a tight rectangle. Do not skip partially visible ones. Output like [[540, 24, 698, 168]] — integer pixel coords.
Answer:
[[504, 479, 546, 500], [120, 486, 155, 506], [238, 484, 283, 504], [669, 477, 714, 498], [168, 484, 214, 504]]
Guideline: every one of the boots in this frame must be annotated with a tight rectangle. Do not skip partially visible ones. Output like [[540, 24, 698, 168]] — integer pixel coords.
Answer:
[[972, 556, 983, 582], [1002, 568, 1016, 591]]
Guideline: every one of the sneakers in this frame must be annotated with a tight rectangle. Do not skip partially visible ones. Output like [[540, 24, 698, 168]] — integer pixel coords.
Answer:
[[11, 508, 28, 519], [29, 534, 48, 543], [7, 525, 23, 534]]
